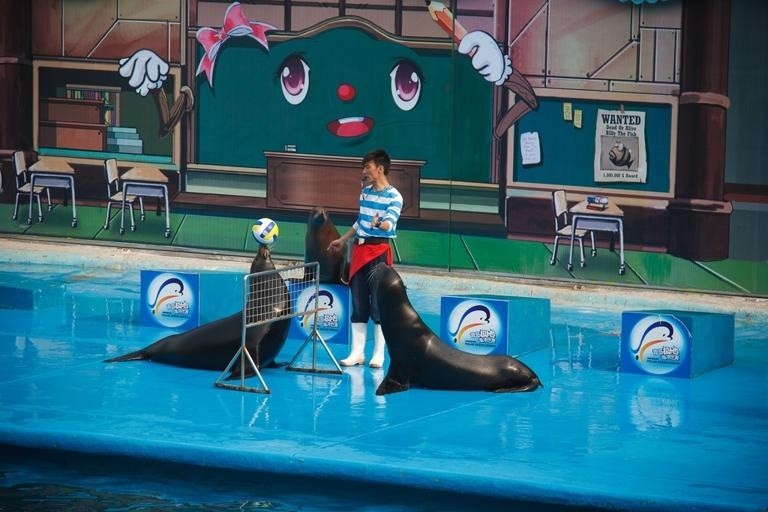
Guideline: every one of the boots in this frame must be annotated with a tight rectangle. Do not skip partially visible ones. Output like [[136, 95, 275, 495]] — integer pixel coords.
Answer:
[[369, 323, 387, 368], [339, 322, 368, 367]]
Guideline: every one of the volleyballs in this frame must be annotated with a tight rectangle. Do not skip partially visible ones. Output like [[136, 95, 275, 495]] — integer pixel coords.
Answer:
[[252, 217, 279, 244]]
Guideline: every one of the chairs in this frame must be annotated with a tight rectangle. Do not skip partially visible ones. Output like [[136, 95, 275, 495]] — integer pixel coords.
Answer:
[[549, 189, 597, 269], [12, 150, 52, 223], [103, 157, 145, 232]]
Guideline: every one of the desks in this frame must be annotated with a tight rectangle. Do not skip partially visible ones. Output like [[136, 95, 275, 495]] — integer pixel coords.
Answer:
[[567, 198, 626, 276], [120, 167, 171, 239], [263, 150, 428, 220], [27, 158, 78, 229]]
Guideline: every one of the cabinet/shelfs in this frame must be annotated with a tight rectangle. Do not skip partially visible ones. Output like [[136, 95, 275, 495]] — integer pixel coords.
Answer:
[[65, 84, 122, 128]]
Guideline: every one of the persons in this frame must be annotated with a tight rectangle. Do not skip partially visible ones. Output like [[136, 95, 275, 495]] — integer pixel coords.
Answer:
[[326, 148, 407, 370]]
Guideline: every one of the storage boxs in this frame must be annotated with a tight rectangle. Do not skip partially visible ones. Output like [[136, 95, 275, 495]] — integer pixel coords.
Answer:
[[38, 97, 108, 153]]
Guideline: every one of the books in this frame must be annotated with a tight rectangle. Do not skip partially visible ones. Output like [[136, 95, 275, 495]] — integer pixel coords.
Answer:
[[585, 193, 609, 212], [70, 89, 117, 128]]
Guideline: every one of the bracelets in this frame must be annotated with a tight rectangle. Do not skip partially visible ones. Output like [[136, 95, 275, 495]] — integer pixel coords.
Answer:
[[374, 219, 382, 228]]
